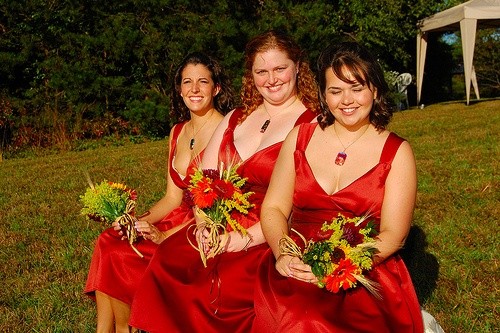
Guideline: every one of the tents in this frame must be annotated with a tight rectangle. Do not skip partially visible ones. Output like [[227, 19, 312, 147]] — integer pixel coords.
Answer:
[[416, 0, 500, 108]]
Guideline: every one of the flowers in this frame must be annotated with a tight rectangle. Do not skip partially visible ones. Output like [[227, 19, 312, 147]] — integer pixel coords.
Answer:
[[279, 203, 407, 302], [79, 173, 151, 259], [183, 144, 255, 268]]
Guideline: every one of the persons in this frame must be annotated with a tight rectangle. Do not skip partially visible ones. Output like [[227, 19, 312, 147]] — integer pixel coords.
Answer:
[[127, 30, 321, 333], [252, 41, 424, 333], [84, 51, 225, 333]]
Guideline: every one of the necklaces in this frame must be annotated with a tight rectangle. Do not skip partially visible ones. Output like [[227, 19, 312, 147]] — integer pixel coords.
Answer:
[[260, 97, 298, 134], [334, 122, 370, 166], [190, 107, 215, 150]]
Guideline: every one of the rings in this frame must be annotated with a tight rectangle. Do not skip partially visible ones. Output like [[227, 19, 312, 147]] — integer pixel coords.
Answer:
[[151, 236, 154, 240], [309, 279, 311, 283]]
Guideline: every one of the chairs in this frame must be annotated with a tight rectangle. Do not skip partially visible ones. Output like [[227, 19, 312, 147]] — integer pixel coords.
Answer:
[[392, 72, 412, 110]]
